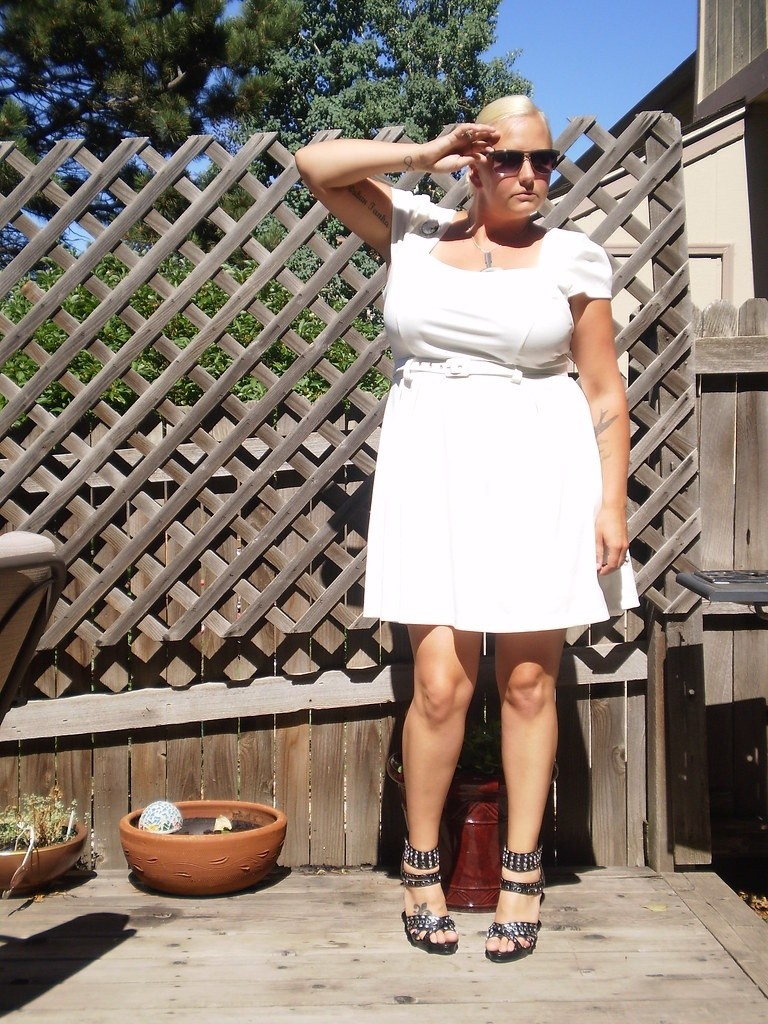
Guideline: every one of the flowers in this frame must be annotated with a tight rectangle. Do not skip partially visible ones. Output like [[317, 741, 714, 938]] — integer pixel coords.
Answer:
[[0, 787, 91, 851]]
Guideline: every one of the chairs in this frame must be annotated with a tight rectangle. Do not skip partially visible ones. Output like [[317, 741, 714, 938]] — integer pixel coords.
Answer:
[[0, 530, 68, 721]]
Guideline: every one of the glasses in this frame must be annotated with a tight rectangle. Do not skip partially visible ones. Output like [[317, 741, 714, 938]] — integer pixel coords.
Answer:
[[483, 147, 560, 176]]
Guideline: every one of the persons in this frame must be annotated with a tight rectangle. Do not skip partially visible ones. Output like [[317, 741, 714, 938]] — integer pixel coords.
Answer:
[[294, 94, 640, 963]]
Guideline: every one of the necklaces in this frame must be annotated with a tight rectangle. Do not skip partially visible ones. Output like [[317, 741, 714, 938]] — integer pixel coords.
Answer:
[[468, 213, 530, 268]]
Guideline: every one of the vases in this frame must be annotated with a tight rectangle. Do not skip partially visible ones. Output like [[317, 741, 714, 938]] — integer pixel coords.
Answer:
[[119, 799, 287, 896], [0, 816, 89, 894]]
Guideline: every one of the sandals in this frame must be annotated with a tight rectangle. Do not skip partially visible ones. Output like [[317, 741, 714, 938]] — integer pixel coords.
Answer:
[[484, 842, 546, 963], [399, 838, 459, 955]]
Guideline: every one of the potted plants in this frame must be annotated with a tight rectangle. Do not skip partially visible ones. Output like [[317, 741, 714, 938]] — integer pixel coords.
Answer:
[[386, 719, 559, 913]]
